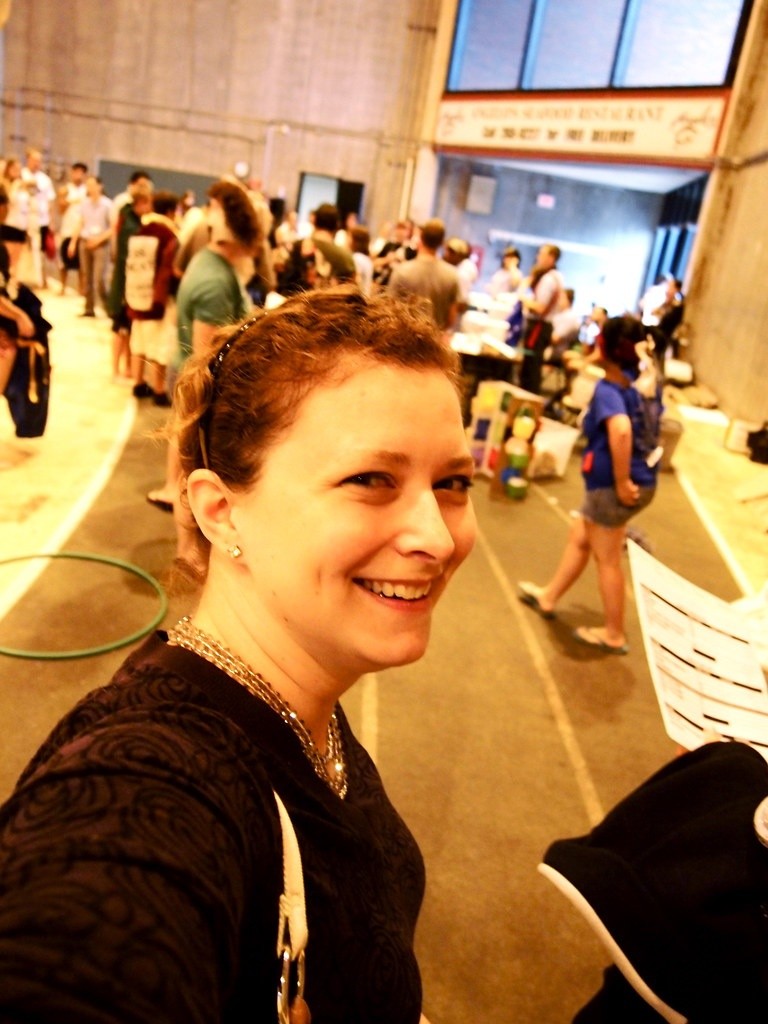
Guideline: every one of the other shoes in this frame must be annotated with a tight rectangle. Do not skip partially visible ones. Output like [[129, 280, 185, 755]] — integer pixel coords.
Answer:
[[154, 392, 172, 407], [146, 490, 174, 514], [133, 381, 154, 398]]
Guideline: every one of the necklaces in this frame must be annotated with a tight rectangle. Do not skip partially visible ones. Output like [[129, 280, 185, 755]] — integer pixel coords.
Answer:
[[166, 615, 348, 800]]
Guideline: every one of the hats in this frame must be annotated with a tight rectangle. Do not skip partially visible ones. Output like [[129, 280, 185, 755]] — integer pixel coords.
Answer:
[[446, 239, 467, 254]]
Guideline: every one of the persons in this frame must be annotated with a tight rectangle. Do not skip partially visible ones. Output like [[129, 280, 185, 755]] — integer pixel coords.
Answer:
[[1, 146, 685, 572], [1, 285, 475, 1023], [518, 317, 658, 654]]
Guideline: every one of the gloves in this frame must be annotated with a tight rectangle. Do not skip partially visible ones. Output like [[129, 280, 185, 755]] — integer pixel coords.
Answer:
[[150, 302, 164, 320]]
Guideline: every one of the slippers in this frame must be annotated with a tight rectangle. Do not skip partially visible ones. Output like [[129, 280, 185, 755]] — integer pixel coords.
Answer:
[[573, 627, 629, 653], [518, 581, 554, 618]]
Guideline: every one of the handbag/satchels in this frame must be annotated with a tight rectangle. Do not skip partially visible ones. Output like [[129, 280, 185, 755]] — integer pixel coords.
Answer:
[[45, 230, 56, 260]]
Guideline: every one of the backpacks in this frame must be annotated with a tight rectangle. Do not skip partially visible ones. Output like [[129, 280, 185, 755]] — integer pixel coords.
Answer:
[[126, 236, 159, 311]]
